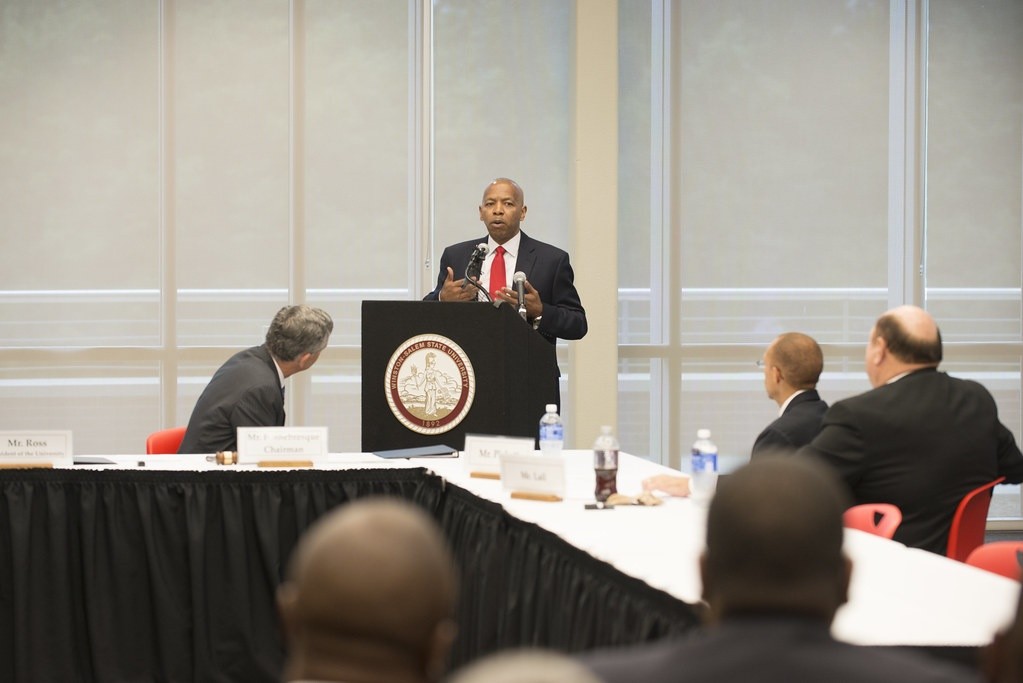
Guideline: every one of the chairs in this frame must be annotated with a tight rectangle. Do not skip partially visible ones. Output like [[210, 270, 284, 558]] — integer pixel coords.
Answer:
[[146, 428, 186, 454], [964, 542, 1023, 580], [843, 504, 903, 540], [947, 477, 1005, 562]]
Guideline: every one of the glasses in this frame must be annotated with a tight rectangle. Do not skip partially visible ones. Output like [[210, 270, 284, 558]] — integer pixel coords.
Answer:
[[757, 358, 784, 379]]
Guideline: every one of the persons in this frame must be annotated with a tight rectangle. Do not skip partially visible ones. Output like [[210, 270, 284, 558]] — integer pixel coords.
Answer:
[[421, 177, 588, 450], [176, 305, 334, 455], [797, 305, 1023, 560], [270, 453, 1023, 683], [751, 331, 830, 461]]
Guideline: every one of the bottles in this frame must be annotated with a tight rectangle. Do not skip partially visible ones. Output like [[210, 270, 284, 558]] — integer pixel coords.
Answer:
[[689, 430, 717, 506], [539, 404, 564, 454], [594, 426, 619, 502]]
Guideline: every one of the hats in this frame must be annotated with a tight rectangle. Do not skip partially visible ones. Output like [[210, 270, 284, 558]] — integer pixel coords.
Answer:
[[706, 455, 848, 618]]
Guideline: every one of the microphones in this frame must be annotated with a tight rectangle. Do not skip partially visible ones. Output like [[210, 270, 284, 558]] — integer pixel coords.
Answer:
[[461, 242, 489, 289], [513, 270, 527, 311]]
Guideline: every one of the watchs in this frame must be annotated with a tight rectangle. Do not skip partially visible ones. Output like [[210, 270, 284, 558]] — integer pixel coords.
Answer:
[[533, 314, 542, 327]]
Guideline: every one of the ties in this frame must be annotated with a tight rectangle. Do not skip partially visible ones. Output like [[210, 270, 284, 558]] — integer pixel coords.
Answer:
[[490, 246, 506, 302]]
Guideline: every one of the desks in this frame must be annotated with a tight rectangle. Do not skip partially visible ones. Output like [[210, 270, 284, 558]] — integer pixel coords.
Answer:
[[410, 450, 1023, 666], [0, 451, 418, 683]]
